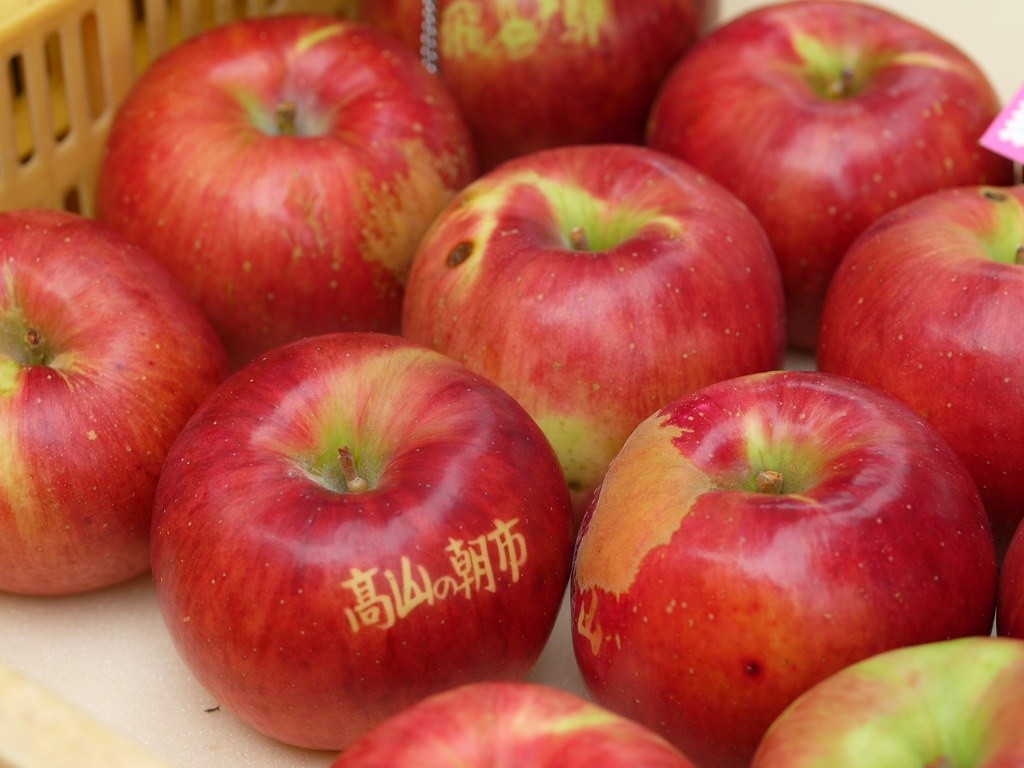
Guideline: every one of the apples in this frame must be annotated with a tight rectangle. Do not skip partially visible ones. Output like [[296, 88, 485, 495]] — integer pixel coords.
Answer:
[[0, 2, 1024, 768]]
